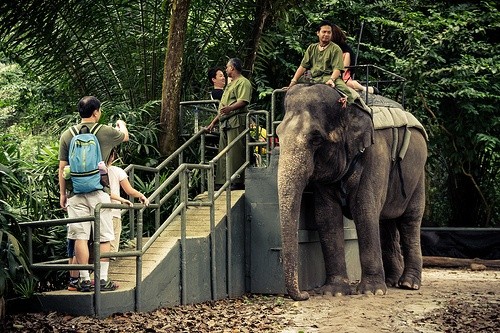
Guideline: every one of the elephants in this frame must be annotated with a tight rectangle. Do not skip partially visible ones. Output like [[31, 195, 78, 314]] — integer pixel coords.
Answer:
[[276, 82, 428, 302]]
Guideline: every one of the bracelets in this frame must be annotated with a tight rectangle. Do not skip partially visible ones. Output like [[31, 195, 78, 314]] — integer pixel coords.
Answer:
[[291, 79, 297, 82]]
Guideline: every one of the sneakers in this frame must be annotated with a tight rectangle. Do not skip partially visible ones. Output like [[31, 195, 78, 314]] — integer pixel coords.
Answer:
[[76, 278, 92, 292], [99, 278, 117, 291]]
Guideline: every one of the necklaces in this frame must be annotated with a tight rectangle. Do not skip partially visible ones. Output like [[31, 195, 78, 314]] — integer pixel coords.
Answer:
[[320, 42, 329, 49]]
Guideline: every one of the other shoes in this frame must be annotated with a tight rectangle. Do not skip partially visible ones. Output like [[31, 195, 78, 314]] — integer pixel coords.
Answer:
[[68, 278, 80, 290]]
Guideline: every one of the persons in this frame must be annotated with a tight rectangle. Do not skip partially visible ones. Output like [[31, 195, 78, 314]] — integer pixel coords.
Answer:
[[249, 122, 268, 167], [58, 95, 129, 291], [331, 24, 364, 92], [66, 224, 94, 291], [210, 68, 226, 112], [206, 58, 252, 193], [94, 147, 148, 291], [282, 21, 353, 104]]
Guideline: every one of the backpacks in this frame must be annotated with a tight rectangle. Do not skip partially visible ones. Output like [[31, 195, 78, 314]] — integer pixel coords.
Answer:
[[67, 123, 109, 194]]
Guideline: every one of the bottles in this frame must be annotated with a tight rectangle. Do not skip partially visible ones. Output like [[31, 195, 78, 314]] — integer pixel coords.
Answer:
[[98, 161, 109, 186], [62, 166, 73, 191]]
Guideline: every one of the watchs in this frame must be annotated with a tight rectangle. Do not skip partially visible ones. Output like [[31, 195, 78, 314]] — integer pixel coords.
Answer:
[[331, 78, 336, 82]]
